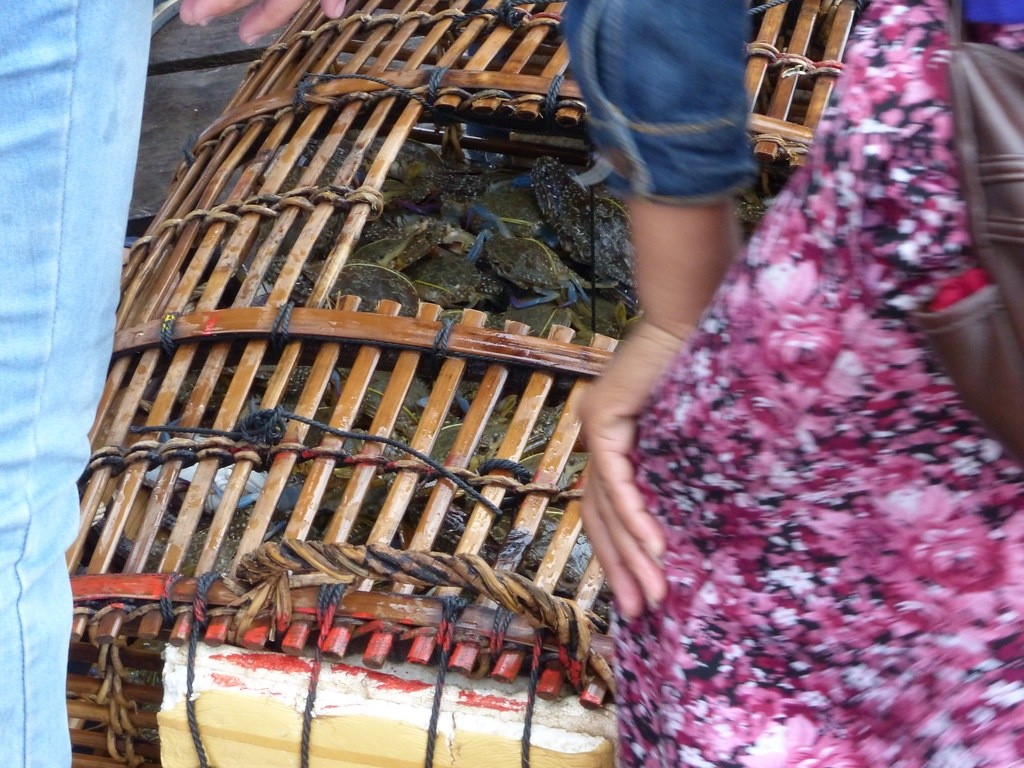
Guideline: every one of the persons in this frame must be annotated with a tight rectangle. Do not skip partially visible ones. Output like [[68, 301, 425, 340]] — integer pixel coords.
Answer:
[[0, 0, 344, 768], [563, 0, 1024, 768]]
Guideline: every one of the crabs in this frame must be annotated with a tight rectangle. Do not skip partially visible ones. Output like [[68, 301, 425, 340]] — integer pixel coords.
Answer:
[[129, 128, 824, 594]]
